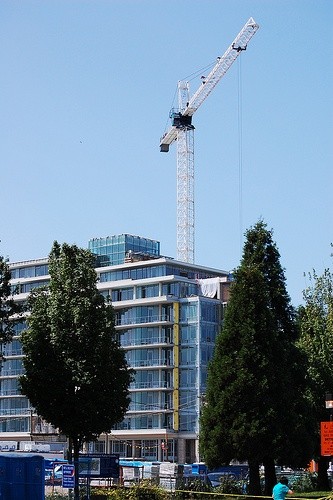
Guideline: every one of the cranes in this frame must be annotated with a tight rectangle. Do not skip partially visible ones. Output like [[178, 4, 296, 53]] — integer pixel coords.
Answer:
[[161, 16, 260, 264]]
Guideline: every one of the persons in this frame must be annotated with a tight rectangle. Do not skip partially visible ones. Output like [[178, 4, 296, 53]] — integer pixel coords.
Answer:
[[271, 476, 294, 500]]
